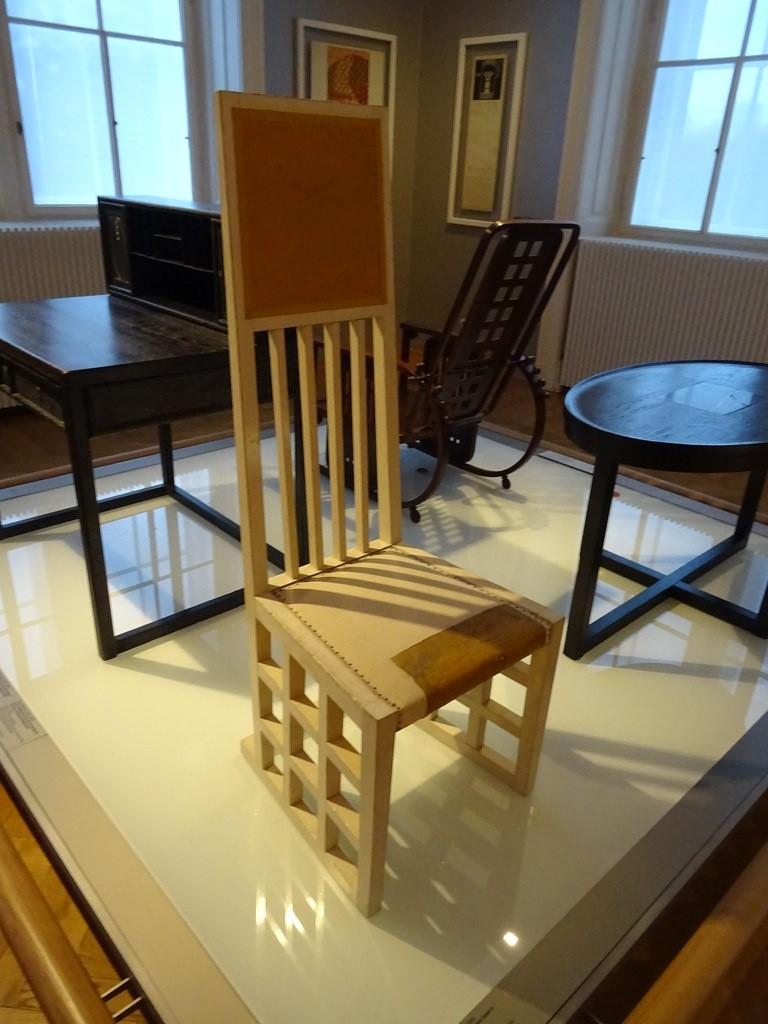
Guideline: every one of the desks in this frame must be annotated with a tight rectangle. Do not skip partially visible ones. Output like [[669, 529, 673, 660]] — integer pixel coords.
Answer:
[[0, 290, 321, 661], [563, 360, 768, 660]]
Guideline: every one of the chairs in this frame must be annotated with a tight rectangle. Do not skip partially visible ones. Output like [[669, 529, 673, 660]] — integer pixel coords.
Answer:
[[314, 220, 580, 522], [215, 90, 565, 915]]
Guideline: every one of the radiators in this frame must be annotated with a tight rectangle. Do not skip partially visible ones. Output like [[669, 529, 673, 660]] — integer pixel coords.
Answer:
[[0, 226, 106, 409], [560, 237, 768, 389]]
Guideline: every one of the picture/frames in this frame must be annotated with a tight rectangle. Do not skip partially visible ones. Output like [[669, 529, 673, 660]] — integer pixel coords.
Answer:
[[295, 19, 397, 181]]
